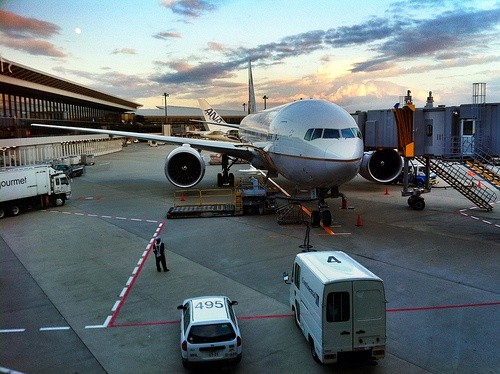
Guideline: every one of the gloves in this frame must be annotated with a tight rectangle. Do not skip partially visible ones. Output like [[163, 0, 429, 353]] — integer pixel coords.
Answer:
[[157, 254, 161, 257]]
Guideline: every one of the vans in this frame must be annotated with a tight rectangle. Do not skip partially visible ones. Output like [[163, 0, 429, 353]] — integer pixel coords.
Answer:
[[283, 250, 388, 364], [39, 153, 95, 177], [390, 158, 437, 188]]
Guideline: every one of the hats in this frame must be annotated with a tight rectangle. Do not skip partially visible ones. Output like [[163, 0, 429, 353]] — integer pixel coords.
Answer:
[[155, 236, 160, 240]]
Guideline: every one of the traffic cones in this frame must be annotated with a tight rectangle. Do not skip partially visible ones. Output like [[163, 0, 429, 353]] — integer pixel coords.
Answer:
[[478, 180, 481, 188], [384, 185, 390, 195], [355, 212, 363, 226], [180, 192, 186, 201]]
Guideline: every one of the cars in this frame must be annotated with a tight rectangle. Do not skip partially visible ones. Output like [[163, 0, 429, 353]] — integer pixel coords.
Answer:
[[177, 294, 241, 369]]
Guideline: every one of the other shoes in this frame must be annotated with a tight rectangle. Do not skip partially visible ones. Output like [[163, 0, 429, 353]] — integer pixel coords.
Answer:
[[157, 269, 161, 272], [164, 269, 169, 272]]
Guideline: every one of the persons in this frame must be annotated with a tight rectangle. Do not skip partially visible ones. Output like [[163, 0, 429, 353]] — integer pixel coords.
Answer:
[[153, 237, 170, 272]]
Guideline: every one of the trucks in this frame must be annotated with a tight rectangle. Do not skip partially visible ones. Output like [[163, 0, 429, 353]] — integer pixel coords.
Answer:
[[0, 164, 73, 217], [166, 175, 277, 224]]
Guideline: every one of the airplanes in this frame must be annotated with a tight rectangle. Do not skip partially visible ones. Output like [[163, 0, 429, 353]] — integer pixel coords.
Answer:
[[31, 59, 403, 227]]
